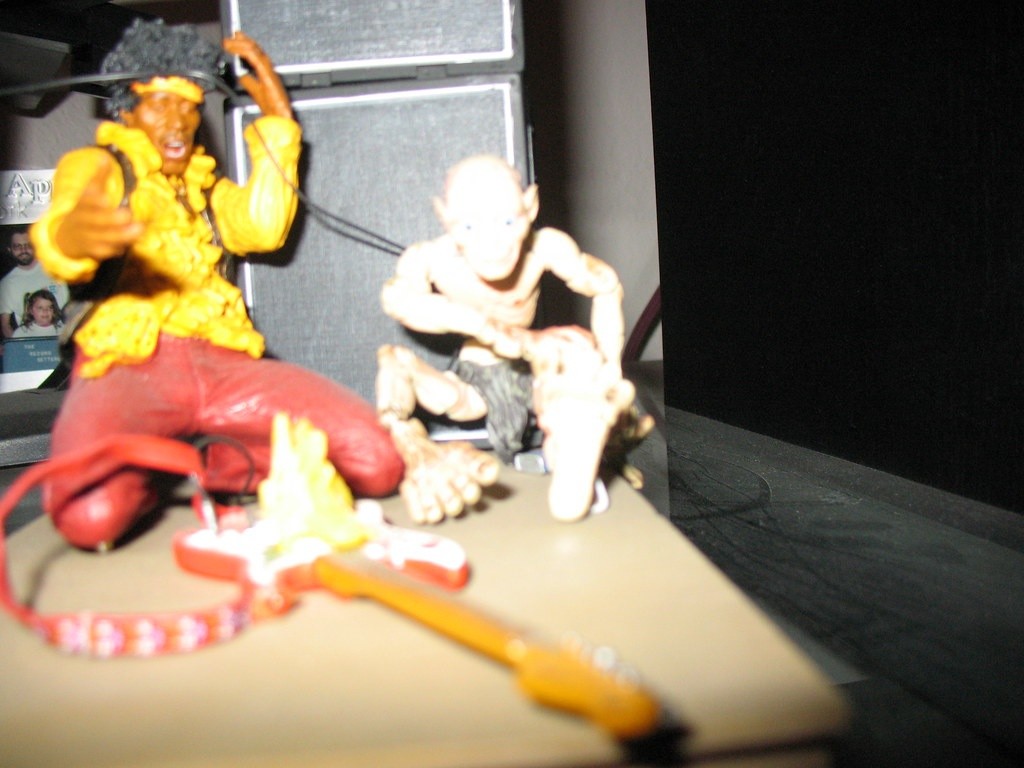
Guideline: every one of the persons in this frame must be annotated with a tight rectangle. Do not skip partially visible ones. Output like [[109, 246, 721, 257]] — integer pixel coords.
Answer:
[[29, 21, 408, 546], [377, 155, 654, 522], [0, 225, 70, 340]]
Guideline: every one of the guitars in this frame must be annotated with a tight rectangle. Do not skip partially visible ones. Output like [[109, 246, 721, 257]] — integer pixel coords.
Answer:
[[174, 408, 669, 747]]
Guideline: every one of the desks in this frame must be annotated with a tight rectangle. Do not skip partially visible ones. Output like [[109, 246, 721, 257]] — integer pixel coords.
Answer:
[[0, 435, 853, 768]]
